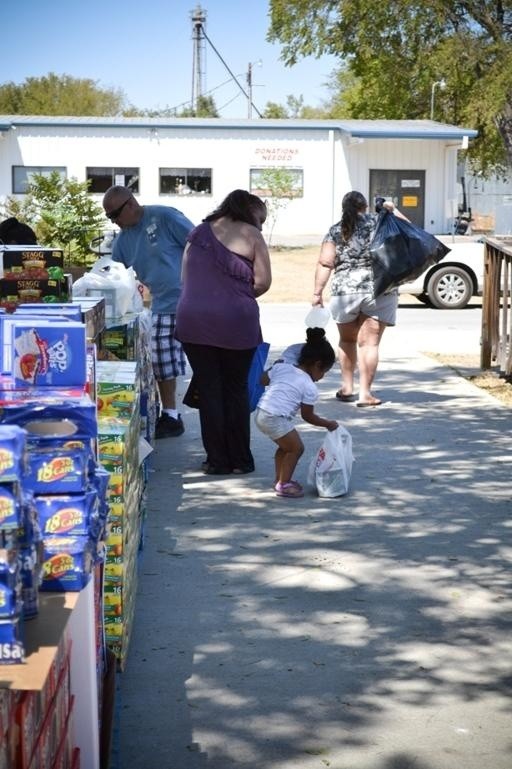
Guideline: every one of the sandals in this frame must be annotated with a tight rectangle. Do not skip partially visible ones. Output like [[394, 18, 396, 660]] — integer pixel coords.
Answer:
[[272, 478, 305, 498]]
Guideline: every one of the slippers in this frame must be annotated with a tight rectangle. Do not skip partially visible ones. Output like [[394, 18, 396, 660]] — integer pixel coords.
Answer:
[[335, 390, 353, 398], [356, 400, 382, 407]]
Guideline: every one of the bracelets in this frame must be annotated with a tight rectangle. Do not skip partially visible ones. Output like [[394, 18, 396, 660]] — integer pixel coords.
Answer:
[[313, 293, 320, 295]]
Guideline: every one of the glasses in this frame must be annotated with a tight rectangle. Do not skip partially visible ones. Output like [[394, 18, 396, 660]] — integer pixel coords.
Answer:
[[105, 197, 132, 219]]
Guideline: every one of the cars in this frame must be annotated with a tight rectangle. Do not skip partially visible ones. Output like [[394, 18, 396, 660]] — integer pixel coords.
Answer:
[[398, 234, 511, 309]]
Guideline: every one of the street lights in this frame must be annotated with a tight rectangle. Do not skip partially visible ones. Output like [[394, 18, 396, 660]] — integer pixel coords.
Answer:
[[431, 77, 447, 119], [246, 59, 263, 118]]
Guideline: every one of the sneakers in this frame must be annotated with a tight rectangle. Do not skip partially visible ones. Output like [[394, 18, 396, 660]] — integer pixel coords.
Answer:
[[201, 461, 248, 475], [154, 411, 185, 440]]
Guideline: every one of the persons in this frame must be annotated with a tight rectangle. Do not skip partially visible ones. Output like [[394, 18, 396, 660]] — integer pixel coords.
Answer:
[[311, 190, 412, 407], [173, 190, 272, 475], [102, 184, 196, 440], [253, 326, 339, 498]]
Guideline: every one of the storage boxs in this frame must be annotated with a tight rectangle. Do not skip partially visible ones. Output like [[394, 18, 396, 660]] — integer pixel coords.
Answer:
[[0, 288, 152, 769]]
